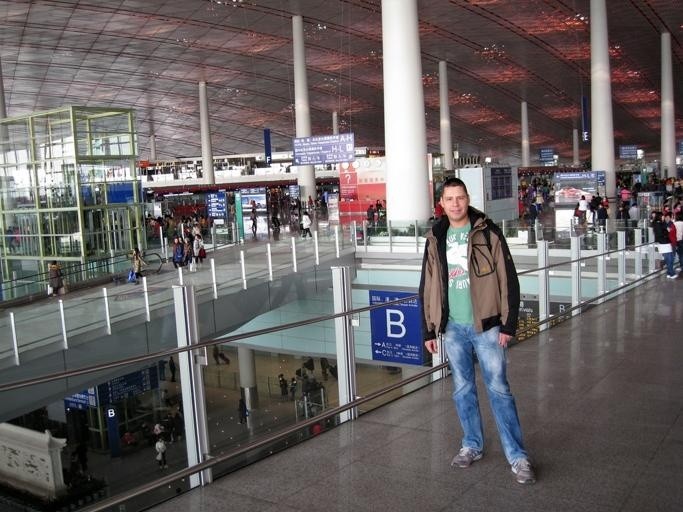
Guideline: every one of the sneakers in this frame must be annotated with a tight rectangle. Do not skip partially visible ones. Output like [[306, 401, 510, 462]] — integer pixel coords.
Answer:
[[451, 446, 484, 467], [511, 457, 536, 485], [667, 274, 679, 279]]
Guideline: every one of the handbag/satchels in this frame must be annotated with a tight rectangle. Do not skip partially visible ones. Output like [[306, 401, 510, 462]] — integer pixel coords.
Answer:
[[48, 284, 54, 295], [128, 270, 137, 283], [176, 254, 183, 261], [576, 210, 583, 216], [198, 248, 206, 258], [300, 224, 305, 230], [246, 409, 249, 416], [156, 452, 162, 460]]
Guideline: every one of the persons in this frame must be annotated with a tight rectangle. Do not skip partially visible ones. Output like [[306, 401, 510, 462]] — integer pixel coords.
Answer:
[[129, 248, 147, 285], [416, 176, 538, 485], [138, 160, 339, 268], [60, 386, 184, 499], [374, 198, 382, 212], [238, 398, 248, 425], [277, 374, 283, 385], [6, 225, 16, 253], [319, 357, 329, 383], [510, 168, 682, 282], [303, 355, 314, 376], [373, 208, 379, 230], [212, 343, 221, 366], [365, 203, 375, 232], [289, 378, 297, 402], [13, 227, 21, 249], [278, 380, 289, 402], [167, 355, 178, 382], [48, 259, 65, 298]]
